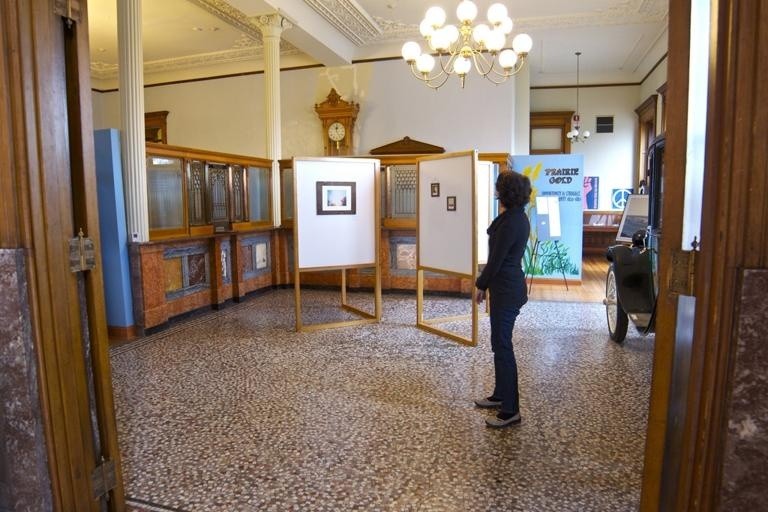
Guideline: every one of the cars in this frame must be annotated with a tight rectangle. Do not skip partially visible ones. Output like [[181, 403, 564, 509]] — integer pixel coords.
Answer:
[[603, 132, 666, 345]]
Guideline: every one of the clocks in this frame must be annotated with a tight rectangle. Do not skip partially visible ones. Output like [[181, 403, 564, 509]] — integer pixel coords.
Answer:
[[313, 87, 361, 157]]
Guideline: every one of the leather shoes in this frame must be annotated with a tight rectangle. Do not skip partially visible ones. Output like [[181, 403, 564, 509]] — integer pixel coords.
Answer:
[[485, 412, 522, 428], [474, 398, 502, 407]]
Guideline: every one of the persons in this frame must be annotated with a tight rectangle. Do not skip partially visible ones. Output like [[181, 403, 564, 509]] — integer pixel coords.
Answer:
[[472, 169, 532, 428]]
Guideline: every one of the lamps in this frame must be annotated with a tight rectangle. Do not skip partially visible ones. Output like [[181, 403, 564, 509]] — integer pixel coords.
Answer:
[[566, 52, 592, 145], [401, 0, 533, 93]]
